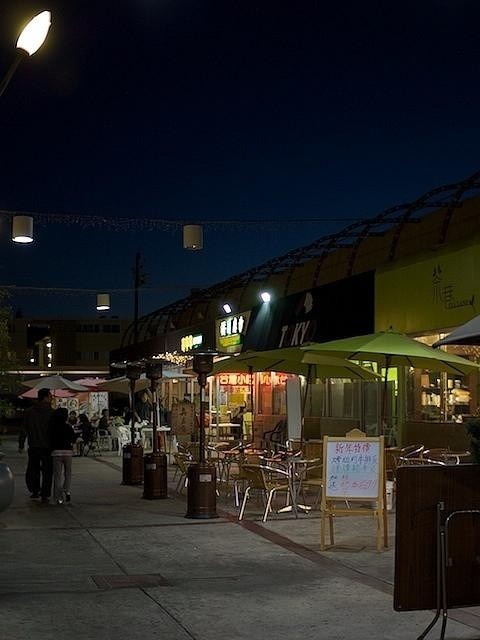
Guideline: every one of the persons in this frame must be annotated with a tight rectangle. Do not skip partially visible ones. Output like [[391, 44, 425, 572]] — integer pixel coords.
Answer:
[[68, 410, 77, 425], [17, 388, 55, 506], [96, 409, 118, 452], [131, 392, 150, 445], [72, 414, 91, 457], [43, 408, 77, 505]]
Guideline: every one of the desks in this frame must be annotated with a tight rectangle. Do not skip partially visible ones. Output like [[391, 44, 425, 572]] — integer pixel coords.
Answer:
[[142, 427, 171, 452], [210, 423, 241, 441], [221, 450, 264, 498], [393, 464, 480, 640]]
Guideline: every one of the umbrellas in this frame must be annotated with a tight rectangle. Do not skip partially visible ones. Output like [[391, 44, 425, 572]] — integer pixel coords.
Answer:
[[97, 370, 195, 444], [301, 325, 480, 435], [432, 314, 480, 349], [248, 342, 384, 449], [205, 351, 262, 441], [20, 374, 89, 407]]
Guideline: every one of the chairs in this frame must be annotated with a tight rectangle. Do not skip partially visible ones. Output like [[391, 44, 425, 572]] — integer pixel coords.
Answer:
[[176, 461, 199, 495], [230, 454, 250, 507], [291, 466, 323, 511], [385, 448, 401, 479], [398, 457, 444, 465], [273, 450, 302, 462], [423, 448, 471, 463], [238, 464, 298, 523], [117, 426, 130, 457], [258, 448, 275, 458], [258, 455, 289, 499], [231, 442, 256, 451], [400, 443, 424, 458], [289, 456, 321, 481], [84, 427, 102, 457], [429, 455, 459, 464], [208, 442, 230, 469], [172, 451, 187, 482], [260, 420, 288, 448], [97, 428, 116, 452], [213, 440, 242, 451], [176, 458, 189, 473]]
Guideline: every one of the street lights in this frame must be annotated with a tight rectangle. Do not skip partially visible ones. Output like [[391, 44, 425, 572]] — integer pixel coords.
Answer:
[[1, 10, 52, 103]]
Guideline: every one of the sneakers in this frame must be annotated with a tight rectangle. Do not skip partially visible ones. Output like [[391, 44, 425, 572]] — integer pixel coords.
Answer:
[[40, 497, 49, 504], [30, 493, 41, 499], [57, 497, 64, 504], [66, 491, 71, 502]]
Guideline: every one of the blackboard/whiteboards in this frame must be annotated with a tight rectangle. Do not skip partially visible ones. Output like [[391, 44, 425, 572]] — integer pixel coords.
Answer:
[[321, 435, 384, 500]]
